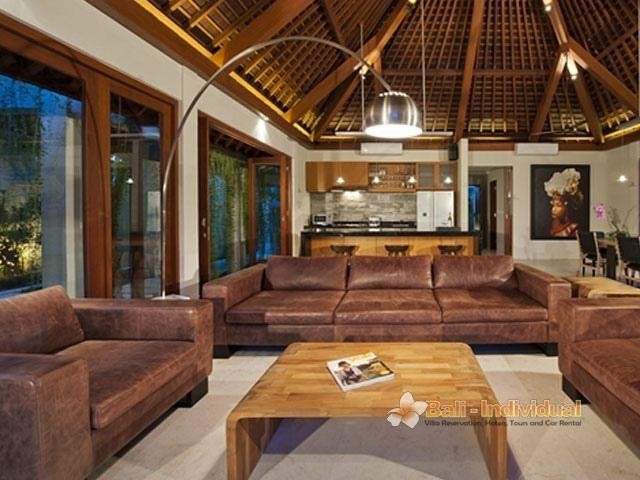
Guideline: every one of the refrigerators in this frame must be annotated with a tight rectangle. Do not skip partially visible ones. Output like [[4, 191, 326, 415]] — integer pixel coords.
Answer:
[[416, 190, 455, 232]]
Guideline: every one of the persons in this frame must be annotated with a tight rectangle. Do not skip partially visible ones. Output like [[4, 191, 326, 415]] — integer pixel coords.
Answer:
[[544, 199, 585, 237], [337, 361, 364, 386]]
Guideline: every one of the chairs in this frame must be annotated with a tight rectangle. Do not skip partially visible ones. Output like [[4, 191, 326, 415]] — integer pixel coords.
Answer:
[[558, 297, 640, 457], [575, 230, 640, 287], [1, 285, 215, 480]]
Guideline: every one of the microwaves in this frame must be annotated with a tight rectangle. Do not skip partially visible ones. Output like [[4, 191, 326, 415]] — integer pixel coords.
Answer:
[[311, 213, 333, 226]]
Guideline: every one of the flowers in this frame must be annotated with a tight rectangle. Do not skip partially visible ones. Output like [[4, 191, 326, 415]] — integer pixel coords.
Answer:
[[591, 200, 638, 240]]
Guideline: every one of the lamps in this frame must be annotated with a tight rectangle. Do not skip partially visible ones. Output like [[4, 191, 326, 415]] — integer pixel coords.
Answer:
[[153, 38, 423, 301]]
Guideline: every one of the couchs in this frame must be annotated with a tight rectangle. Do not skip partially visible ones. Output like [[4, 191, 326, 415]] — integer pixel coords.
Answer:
[[203, 256, 571, 358]]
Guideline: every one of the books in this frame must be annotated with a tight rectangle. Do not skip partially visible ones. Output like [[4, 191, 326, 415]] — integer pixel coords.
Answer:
[[325, 352, 397, 393]]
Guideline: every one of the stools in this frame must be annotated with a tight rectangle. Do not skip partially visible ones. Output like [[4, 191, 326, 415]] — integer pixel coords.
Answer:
[[330, 245, 463, 256]]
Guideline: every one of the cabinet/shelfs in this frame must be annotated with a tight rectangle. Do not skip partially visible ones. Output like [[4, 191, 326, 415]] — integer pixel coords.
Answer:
[[305, 161, 457, 193]]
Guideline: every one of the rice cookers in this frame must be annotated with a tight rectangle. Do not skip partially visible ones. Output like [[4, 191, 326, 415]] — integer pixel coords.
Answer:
[[368, 215, 381, 228]]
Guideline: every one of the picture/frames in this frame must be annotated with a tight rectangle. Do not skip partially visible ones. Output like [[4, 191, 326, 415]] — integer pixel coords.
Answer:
[[531, 164, 589, 239]]
